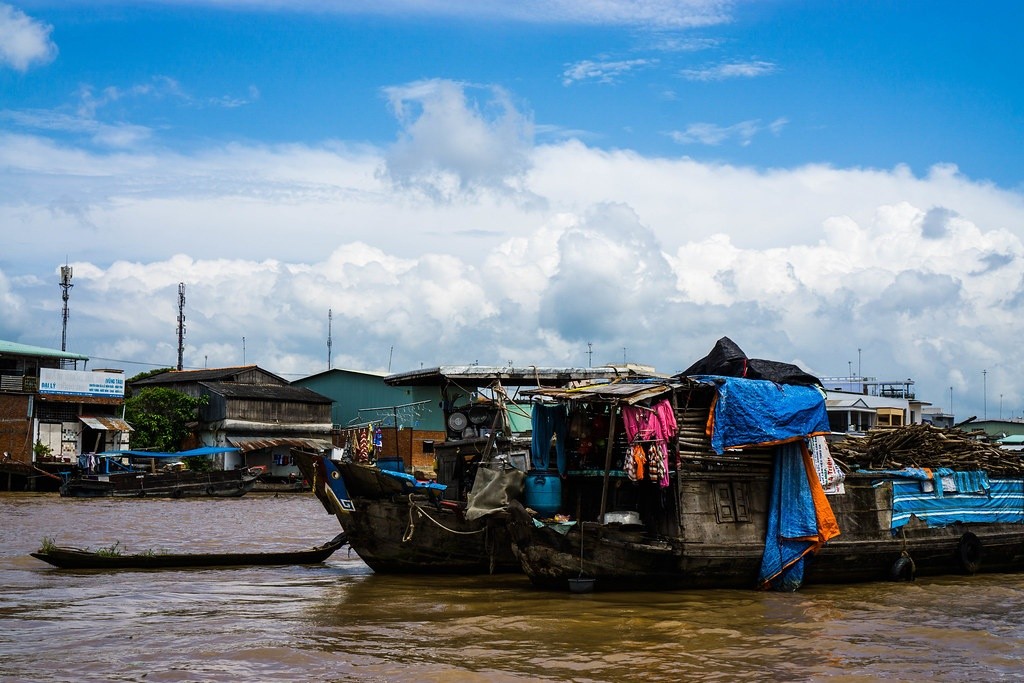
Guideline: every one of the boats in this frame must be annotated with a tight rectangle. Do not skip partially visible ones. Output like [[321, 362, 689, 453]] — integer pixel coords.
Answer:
[[59, 448, 266, 499], [30, 535, 348, 570], [289, 374, 1023, 584]]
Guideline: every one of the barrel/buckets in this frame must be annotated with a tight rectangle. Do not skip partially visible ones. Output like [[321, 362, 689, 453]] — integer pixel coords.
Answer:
[[568, 578, 595, 592], [526, 469, 562, 518]]
